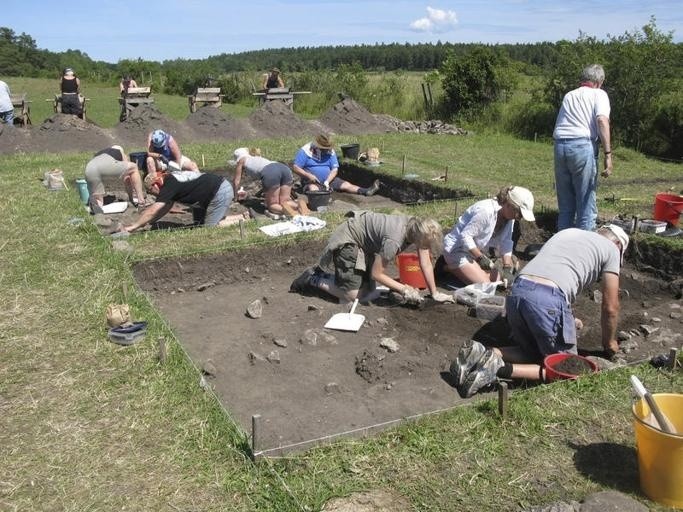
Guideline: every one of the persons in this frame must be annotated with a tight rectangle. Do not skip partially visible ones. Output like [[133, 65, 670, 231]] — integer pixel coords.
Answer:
[[118, 71, 137, 96], [0, 78, 15, 130], [232, 146, 310, 220], [441, 185, 533, 288], [144, 128, 201, 177], [451, 224, 626, 398], [112, 171, 257, 232], [263, 68, 283, 92], [58, 68, 84, 117], [551, 63, 612, 233], [290, 133, 380, 197], [288, 213, 454, 306], [84, 144, 150, 215]]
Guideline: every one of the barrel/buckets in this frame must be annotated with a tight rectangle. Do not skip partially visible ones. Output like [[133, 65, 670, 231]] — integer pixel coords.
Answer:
[[544, 353, 597, 384], [129, 152, 147, 170], [631, 392, 683, 509], [75, 179, 90, 204], [655, 193, 683, 224], [367, 147, 379, 162], [44, 170, 64, 189], [306, 191, 330, 211], [398, 253, 433, 289], [340, 144, 359, 160]]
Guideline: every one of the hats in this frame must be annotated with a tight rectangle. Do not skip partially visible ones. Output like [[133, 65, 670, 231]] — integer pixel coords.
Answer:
[[314, 134, 333, 150], [601, 223, 629, 267], [151, 130, 166, 148], [507, 186, 536, 222], [234, 148, 250, 163]]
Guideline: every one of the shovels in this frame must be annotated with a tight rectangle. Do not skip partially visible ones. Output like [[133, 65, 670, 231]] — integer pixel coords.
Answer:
[[323, 298, 366, 332]]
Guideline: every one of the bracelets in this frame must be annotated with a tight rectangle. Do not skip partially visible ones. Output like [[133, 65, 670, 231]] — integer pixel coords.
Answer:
[[602, 149, 611, 157]]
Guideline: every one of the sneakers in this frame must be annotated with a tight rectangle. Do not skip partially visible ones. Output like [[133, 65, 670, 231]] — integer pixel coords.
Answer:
[[450, 340, 505, 398], [290, 263, 324, 290]]
